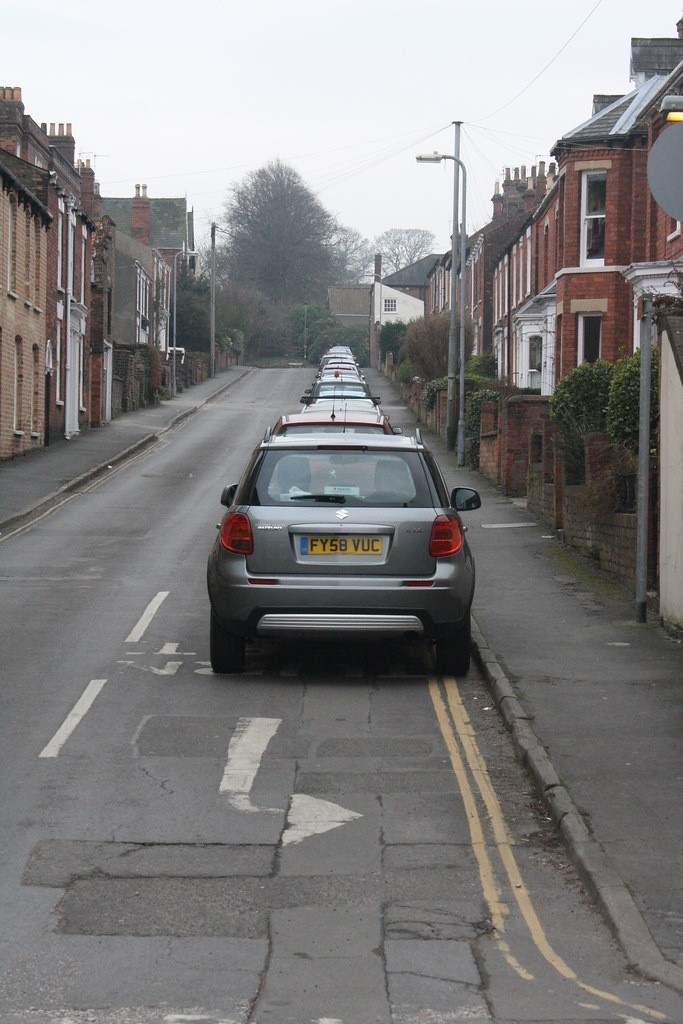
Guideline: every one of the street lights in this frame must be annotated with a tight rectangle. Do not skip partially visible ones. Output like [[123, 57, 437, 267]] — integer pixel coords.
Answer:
[[414, 152, 467, 467], [365, 274, 383, 375], [301, 301, 309, 367], [173, 251, 200, 396]]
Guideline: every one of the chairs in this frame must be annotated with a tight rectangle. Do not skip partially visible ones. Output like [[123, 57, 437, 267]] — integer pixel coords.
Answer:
[[363, 459, 413, 502], [275, 456, 312, 494]]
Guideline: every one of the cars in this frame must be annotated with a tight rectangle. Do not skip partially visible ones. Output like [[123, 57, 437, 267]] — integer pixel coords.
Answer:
[[263, 346, 408, 498]]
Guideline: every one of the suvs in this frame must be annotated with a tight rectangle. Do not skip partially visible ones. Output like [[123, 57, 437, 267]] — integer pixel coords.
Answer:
[[206, 426, 482, 676]]
